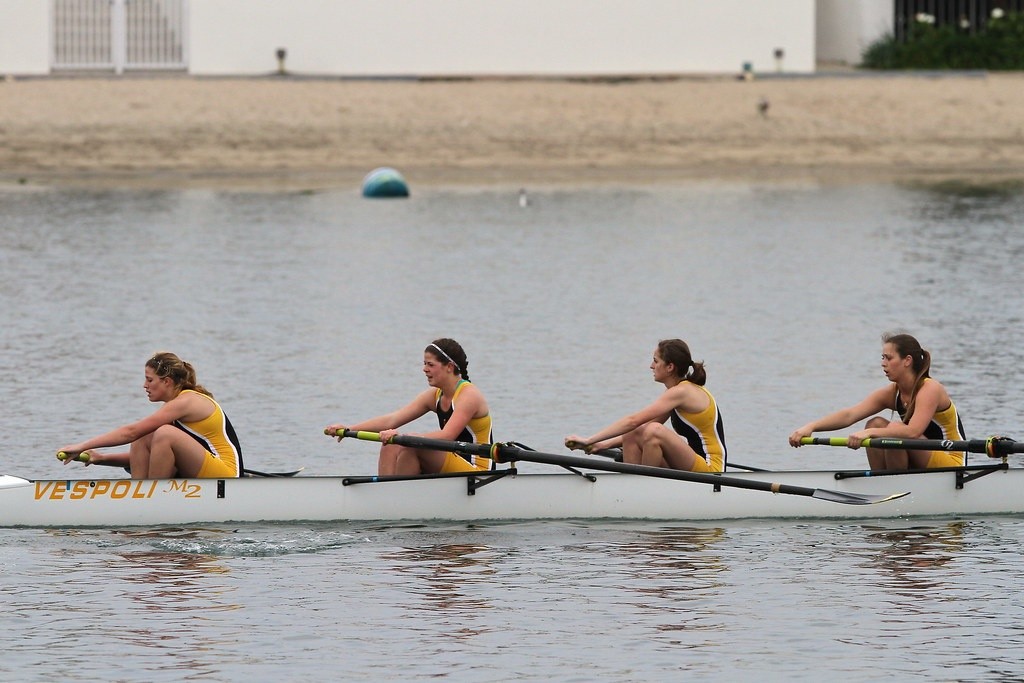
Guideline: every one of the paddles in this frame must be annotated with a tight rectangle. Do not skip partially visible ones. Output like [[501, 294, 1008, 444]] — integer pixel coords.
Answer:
[[798, 435, 1024, 459], [562, 433, 778, 476], [321, 420, 911, 507], [55, 451, 307, 479]]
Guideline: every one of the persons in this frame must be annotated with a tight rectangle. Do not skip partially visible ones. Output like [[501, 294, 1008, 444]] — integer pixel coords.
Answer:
[[56, 352, 244, 480], [789, 334, 968, 470], [565, 339, 727, 473], [326, 338, 496, 476]]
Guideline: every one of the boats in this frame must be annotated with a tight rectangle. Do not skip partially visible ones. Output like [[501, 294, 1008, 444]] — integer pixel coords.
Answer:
[[1, 467, 1024, 522]]
